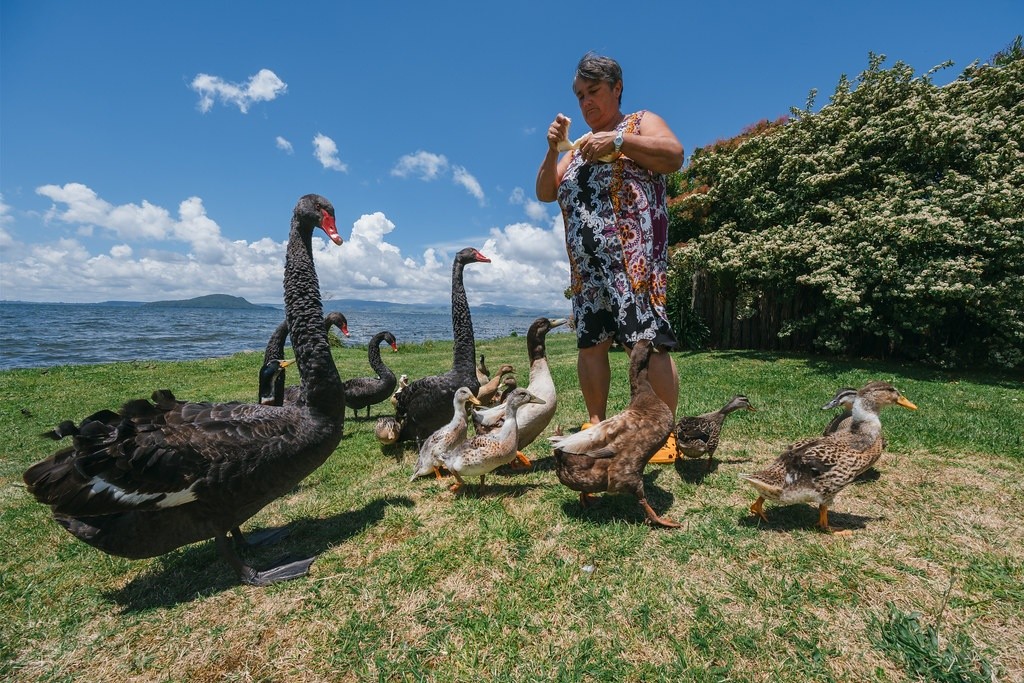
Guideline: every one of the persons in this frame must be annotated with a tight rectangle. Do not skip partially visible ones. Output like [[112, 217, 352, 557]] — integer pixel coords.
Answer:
[[536, 50, 685, 463]]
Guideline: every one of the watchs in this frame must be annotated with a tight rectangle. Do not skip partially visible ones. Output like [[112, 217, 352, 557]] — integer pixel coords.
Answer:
[[614, 129, 624, 153]]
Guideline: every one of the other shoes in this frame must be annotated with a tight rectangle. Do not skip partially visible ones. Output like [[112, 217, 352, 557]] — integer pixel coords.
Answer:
[[580, 422, 592, 430], [647, 432, 678, 463]]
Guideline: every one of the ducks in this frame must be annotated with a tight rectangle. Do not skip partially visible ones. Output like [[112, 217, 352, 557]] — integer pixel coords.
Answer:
[[22, 192, 921, 586]]
